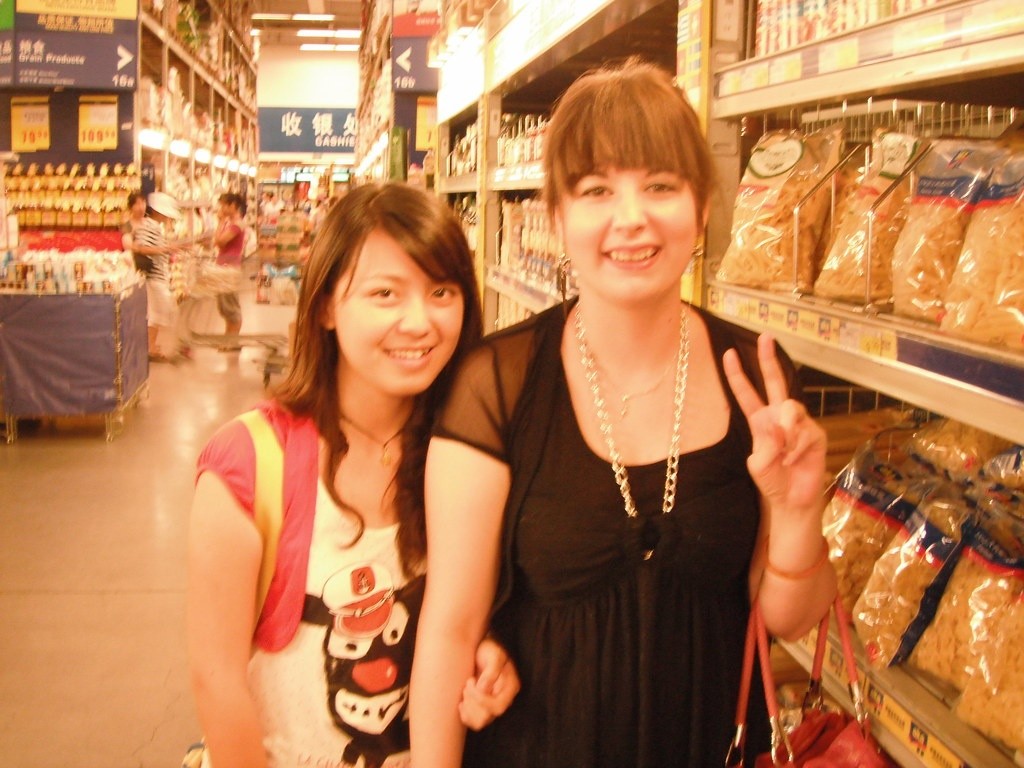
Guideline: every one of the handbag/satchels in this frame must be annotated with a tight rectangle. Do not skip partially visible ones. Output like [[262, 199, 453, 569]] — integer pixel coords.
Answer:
[[730, 566, 892, 767], [175, 735, 214, 768]]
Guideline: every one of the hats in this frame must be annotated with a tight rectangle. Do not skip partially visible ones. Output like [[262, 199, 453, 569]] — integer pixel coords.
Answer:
[[149, 190, 183, 221]]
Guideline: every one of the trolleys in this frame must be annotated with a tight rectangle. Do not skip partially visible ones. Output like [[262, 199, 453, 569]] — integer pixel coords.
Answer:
[[166, 243, 289, 391]]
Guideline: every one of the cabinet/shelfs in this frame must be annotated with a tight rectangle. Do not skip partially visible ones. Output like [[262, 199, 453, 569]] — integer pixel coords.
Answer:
[[2, 1, 1024, 768]]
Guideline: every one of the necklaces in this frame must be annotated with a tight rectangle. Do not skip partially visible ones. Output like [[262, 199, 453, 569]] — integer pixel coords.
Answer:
[[340, 408, 415, 469], [589, 347, 682, 420], [572, 301, 692, 562]]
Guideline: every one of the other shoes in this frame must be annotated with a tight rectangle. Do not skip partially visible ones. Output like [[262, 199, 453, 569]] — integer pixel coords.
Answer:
[[146, 351, 174, 365], [218, 342, 246, 354]]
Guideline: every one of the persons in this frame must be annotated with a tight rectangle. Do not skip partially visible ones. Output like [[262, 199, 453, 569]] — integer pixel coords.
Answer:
[[212, 192, 247, 353], [185, 181, 522, 768], [258, 188, 338, 248], [120, 192, 153, 273], [131, 192, 193, 364], [408, 52, 837, 768]]
